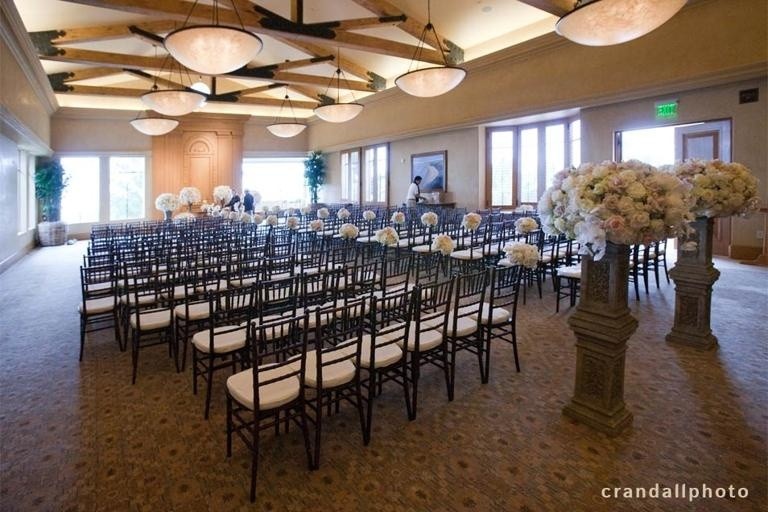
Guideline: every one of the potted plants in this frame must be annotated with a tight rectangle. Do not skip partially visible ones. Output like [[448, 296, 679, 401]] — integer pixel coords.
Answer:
[[33, 161, 70, 249]]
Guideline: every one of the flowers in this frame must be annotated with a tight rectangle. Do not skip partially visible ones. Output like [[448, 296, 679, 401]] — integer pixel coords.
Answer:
[[179, 186, 201, 214], [537, 158, 694, 262], [212, 186, 234, 209], [657, 160, 762, 224], [154, 192, 179, 213]]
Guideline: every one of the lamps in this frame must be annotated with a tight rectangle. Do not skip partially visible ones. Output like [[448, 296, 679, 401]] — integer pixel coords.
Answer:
[[164, 2, 264, 78], [393, 2, 468, 100], [264, 59, 308, 140], [553, 0, 690, 51], [137, 21, 208, 119], [311, 46, 365, 126], [129, 110, 180, 139]]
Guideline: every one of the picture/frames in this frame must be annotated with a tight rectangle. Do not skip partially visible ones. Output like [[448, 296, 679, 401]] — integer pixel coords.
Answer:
[[410, 150, 450, 196]]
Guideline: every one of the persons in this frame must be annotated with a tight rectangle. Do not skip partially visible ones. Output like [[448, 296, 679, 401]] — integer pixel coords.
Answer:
[[224, 189, 241, 212], [242, 189, 254, 214], [406, 176, 427, 208]]
